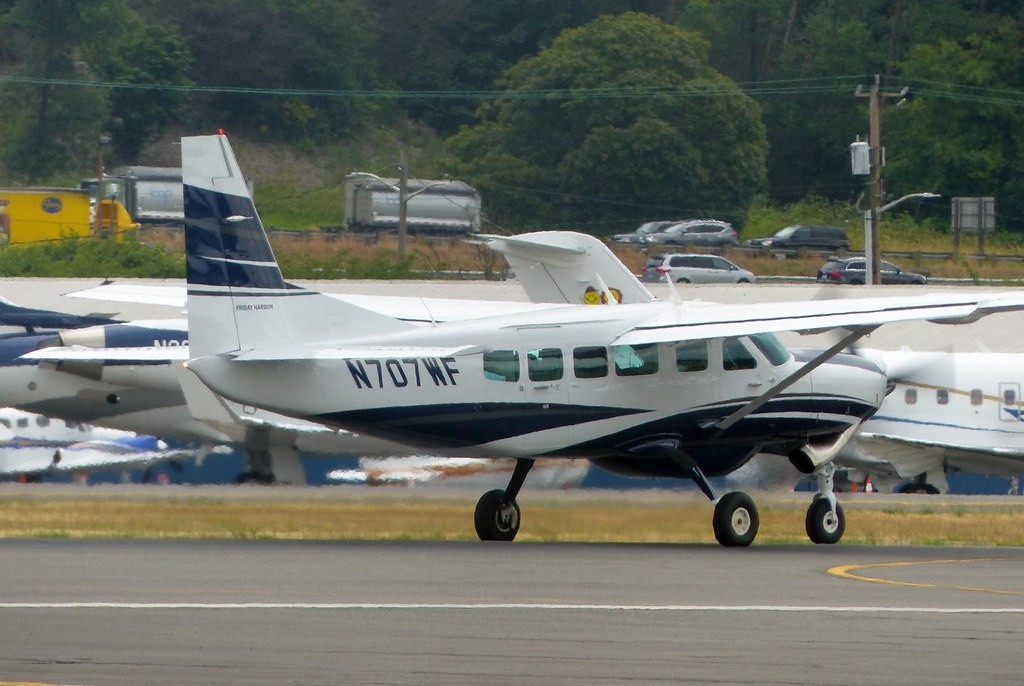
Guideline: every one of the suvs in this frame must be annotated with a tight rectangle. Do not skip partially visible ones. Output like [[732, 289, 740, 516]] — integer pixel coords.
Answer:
[[643, 218, 738, 249], [608, 220, 675, 245], [816, 254, 928, 285], [746, 224, 850, 260]]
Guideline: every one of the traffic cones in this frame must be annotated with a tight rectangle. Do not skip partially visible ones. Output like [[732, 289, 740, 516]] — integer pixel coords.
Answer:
[[863, 476, 874, 492]]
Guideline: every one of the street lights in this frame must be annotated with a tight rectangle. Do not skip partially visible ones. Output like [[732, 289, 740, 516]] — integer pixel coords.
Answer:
[[865, 193, 942, 285], [345, 169, 449, 264], [96, 136, 112, 239]]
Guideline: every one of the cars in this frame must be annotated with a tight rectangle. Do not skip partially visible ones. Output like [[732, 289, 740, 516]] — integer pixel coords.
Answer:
[[791, 467, 878, 492]]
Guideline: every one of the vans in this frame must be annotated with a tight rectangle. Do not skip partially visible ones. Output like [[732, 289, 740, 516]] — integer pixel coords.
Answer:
[[641, 251, 756, 284]]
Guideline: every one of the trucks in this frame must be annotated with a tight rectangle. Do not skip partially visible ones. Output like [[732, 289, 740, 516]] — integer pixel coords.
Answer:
[[0, 188, 142, 246], [80, 165, 185, 224]]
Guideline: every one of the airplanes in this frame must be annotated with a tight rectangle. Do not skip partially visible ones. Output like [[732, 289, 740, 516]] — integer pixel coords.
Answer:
[[0, 128, 1024, 548]]
